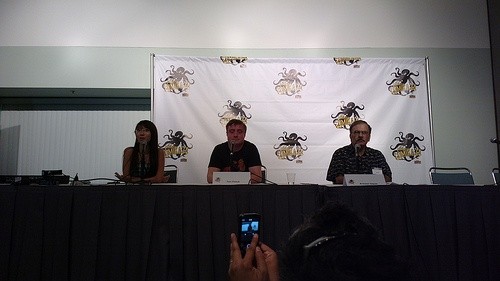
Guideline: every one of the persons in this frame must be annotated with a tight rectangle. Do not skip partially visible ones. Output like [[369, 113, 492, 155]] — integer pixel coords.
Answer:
[[326, 120, 392, 182], [207, 119, 262, 184], [114, 120, 170, 183], [227, 199, 411, 281]]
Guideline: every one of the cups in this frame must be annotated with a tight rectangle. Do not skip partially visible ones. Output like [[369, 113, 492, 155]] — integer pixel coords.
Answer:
[[286, 173, 296, 185]]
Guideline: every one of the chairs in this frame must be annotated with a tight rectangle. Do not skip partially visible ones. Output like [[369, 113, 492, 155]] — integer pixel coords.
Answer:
[[164, 165, 178, 183], [429, 167, 474, 184]]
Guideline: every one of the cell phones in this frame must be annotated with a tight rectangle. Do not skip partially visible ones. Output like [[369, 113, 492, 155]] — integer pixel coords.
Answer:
[[239, 213, 263, 260]]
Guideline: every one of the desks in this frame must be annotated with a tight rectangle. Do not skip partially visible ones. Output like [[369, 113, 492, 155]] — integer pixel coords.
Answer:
[[1, 183, 500, 281]]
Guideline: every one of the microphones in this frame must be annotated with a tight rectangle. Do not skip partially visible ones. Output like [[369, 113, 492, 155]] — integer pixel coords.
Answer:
[[139, 142, 146, 154], [355, 144, 361, 159], [490, 137, 500, 144], [230, 140, 235, 155]]
